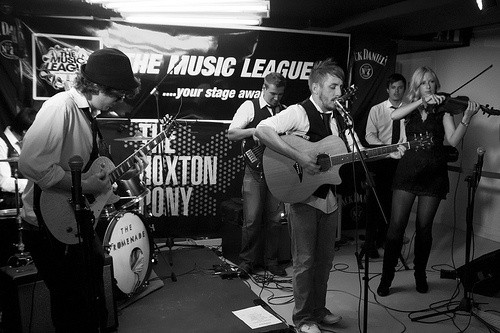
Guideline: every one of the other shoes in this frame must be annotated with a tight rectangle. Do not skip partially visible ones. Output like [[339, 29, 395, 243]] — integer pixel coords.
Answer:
[[240, 265, 255, 279], [365, 242, 380, 259], [266, 265, 286, 277]]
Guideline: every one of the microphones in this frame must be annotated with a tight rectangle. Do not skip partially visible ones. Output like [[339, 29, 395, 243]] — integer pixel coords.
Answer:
[[67, 155, 84, 213], [476, 146, 486, 182]]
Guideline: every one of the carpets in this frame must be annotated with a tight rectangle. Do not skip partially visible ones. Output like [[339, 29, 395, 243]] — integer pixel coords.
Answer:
[[117, 245, 289, 333]]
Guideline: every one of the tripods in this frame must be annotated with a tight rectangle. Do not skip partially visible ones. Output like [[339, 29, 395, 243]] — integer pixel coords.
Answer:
[[411, 172, 500, 333], [133, 57, 204, 283]]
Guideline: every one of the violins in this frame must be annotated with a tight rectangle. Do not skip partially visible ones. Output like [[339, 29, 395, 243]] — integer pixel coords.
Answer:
[[425, 92, 500, 118]]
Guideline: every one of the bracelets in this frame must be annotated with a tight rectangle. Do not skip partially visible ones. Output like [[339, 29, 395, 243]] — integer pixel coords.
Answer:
[[421, 96, 428, 108]]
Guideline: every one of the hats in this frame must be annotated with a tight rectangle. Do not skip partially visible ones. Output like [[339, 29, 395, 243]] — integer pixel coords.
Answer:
[[81, 48, 139, 91]]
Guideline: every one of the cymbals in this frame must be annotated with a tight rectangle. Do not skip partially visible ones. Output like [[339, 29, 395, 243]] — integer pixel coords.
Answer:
[[0, 158, 19, 162], [113, 135, 153, 141]]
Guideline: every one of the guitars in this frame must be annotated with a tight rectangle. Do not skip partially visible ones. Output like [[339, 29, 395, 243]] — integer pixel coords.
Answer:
[[240, 83, 359, 171], [264, 131, 437, 204], [39, 113, 182, 246]]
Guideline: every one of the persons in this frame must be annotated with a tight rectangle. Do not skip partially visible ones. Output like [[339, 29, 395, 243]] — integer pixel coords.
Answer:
[[229, 71, 289, 278], [0, 106, 37, 256], [255, 61, 407, 333], [363, 74, 408, 259], [376, 65, 481, 297], [17, 48, 148, 333]]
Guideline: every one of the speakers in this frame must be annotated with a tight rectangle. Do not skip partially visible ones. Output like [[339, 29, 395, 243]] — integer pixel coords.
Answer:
[[3, 251, 119, 333], [222, 217, 291, 266]]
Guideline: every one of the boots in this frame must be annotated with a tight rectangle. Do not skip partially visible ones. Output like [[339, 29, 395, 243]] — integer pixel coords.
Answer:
[[377, 239, 403, 297], [414, 237, 432, 293]]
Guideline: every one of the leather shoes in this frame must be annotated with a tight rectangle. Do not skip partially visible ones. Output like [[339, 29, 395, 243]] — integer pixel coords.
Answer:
[[299, 323, 320, 333], [311, 310, 341, 324]]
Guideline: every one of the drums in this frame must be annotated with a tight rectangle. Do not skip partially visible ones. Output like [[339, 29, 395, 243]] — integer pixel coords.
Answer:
[[1, 209, 37, 267], [101, 204, 116, 215], [99, 211, 155, 301], [114, 165, 148, 211]]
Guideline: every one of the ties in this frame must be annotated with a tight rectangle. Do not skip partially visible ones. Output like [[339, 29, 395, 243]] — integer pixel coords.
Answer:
[[390, 106, 400, 144], [322, 113, 332, 134], [266, 106, 277, 117]]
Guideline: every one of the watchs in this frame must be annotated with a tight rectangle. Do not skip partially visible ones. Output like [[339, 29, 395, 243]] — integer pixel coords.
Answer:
[[460, 120, 469, 128]]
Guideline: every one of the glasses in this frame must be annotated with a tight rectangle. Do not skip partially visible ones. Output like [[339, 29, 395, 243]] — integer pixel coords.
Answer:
[[112, 93, 126, 104]]
